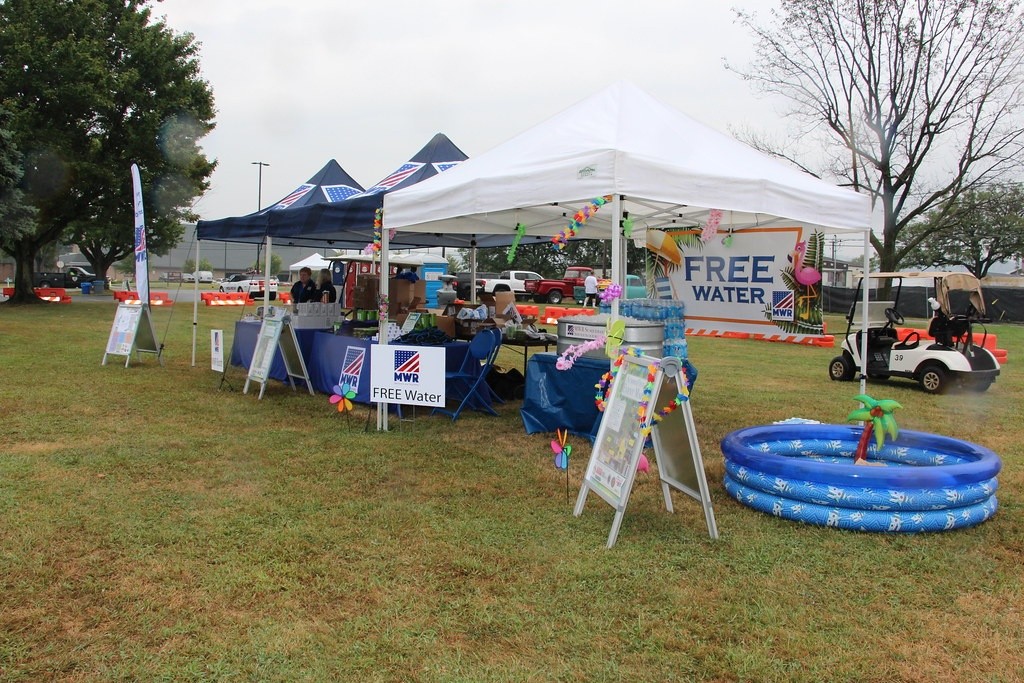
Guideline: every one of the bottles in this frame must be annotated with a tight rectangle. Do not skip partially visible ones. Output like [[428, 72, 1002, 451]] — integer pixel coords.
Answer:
[[457, 303, 488, 321], [599, 298, 686, 363]]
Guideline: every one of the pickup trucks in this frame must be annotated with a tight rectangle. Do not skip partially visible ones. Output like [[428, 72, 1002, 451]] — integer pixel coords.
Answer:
[[573, 274, 647, 306], [524, 266, 605, 304], [32, 267, 110, 289], [476, 271, 546, 301]]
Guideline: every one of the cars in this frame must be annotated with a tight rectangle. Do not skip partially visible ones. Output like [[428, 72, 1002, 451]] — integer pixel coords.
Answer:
[[270, 276, 279, 285], [182, 274, 195, 283]]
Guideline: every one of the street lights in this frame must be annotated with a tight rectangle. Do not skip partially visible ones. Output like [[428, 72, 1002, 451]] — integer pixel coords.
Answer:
[[252, 162, 270, 273]]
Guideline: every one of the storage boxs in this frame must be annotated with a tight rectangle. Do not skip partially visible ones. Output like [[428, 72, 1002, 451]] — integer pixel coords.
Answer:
[[353, 273, 455, 339]]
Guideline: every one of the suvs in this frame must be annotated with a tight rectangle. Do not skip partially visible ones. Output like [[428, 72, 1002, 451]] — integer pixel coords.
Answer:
[[219, 274, 277, 301], [439, 270, 503, 301]]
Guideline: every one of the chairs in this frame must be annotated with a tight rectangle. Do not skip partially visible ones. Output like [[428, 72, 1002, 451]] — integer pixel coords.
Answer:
[[429, 326, 503, 422]]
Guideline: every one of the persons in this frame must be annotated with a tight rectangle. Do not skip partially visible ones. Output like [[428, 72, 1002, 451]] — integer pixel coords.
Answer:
[[391, 267, 406, 279], [583, 270, 598, 308], [313, 269, 336, 303], [405, 266, 419, 283], [289, 267, 316, 303]]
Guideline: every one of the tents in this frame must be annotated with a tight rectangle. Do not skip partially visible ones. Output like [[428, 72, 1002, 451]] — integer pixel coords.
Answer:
[[290, 252, 334, 282], [190, 132, 588, 368], [375, 78, 873, 431]]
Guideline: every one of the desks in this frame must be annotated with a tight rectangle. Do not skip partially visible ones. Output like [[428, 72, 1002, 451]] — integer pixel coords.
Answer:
[[233, 321, 331, 386], [306, 330, 493, 413], [455, 331, 558, 380], [518, 351, 699, 451]]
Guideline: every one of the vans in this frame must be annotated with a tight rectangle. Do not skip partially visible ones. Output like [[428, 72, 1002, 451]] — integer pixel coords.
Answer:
[[159, 272, 182, 283], [193, 271, 213, 283]]
